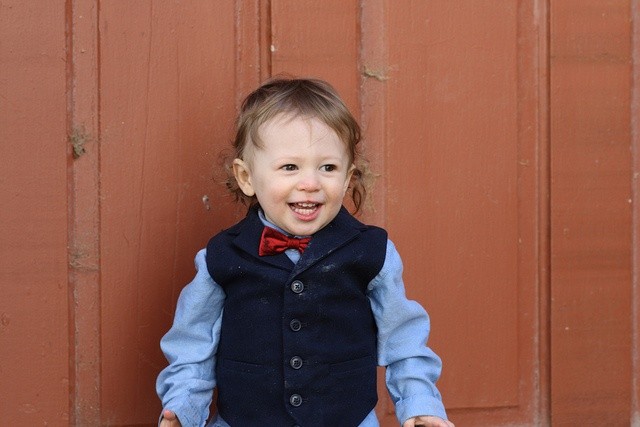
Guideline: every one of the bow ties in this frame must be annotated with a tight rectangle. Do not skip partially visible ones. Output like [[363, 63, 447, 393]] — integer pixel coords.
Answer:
[[258, 226, 311, 257]]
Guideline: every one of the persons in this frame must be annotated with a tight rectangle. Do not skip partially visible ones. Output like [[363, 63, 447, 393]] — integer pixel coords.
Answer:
[[155, 77, 456, 427]]
